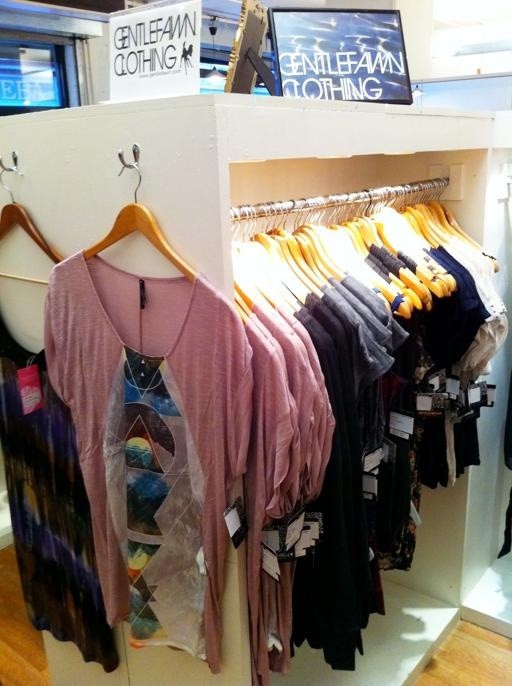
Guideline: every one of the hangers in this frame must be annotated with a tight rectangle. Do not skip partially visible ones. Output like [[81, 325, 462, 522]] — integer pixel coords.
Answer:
[[230, 175, 499, 351], [84, 162, 195, 286], [0, 204, 64, 286]]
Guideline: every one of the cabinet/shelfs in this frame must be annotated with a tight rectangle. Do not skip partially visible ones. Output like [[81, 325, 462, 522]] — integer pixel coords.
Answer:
[[0, 96, 491, 686]]
